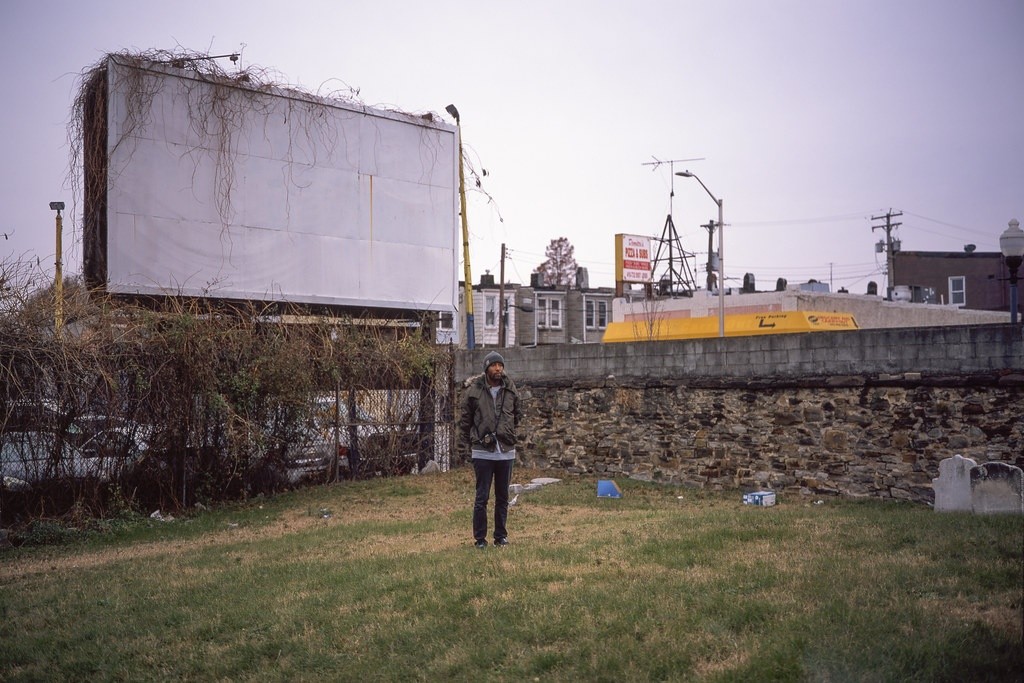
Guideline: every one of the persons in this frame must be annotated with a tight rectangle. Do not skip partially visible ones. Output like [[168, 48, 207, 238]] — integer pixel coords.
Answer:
[[458, 351, 522, 548]]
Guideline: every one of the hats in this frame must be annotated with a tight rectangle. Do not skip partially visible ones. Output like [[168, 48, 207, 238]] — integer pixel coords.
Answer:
[[483, 351, 504, 373]]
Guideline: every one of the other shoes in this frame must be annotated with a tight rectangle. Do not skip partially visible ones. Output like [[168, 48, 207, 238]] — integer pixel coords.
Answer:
[[475, 540, 487, 549], [493, 538, 510, 547]]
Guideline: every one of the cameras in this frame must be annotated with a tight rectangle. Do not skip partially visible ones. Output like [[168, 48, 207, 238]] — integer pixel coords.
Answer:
[[482, 433, 496, 448]]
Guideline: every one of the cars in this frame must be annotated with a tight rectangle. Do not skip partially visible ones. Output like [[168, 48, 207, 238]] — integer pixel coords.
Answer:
[[1, 399, 418, 507]]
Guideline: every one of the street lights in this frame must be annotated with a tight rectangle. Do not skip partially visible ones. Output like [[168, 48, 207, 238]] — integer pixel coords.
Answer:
[[675, 169, 724, 337], [446, 104, 476, 353], [50, 202, 67, 401], [999, 219, 1024, 322]]
